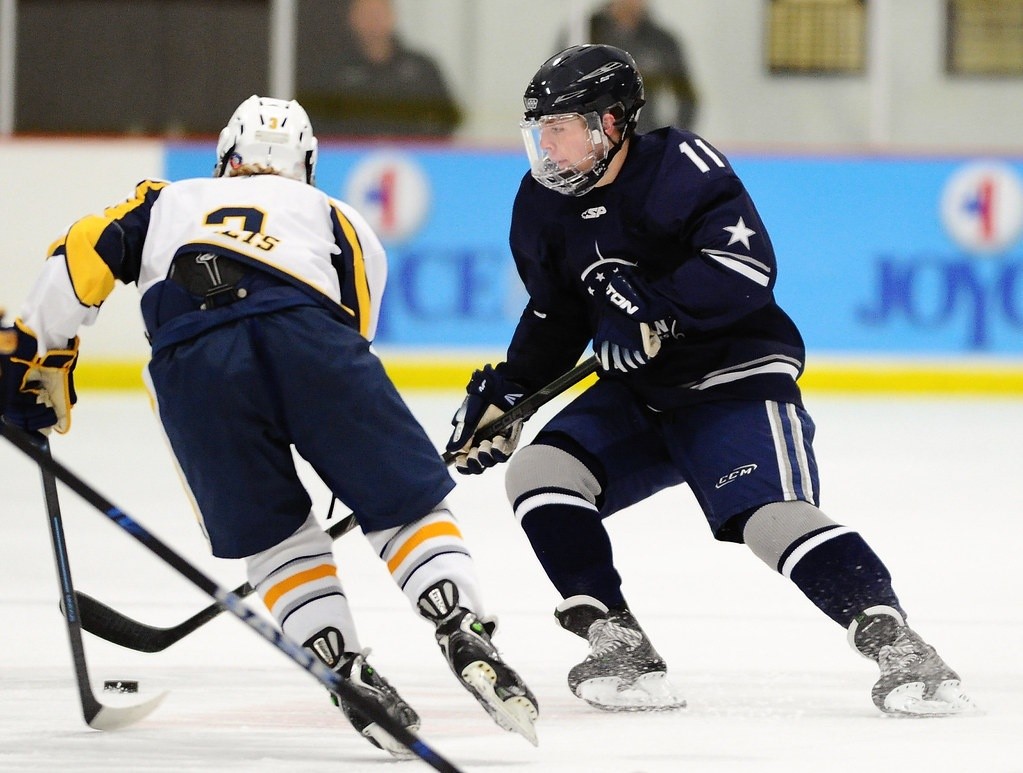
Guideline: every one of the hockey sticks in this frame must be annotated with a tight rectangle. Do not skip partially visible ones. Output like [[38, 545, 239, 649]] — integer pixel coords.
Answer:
[[33, 427, 170, 733], [0, 416, 461, 772], [57, 327, 661, 654]]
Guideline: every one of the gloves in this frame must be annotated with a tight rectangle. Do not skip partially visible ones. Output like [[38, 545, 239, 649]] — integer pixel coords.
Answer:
[[0, 316, 76, 434], [446, 364, 534, 476], [593, 267, 670, 377]]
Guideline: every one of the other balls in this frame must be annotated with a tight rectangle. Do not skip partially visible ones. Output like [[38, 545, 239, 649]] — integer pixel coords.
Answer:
[[103, 680, 139, 694]]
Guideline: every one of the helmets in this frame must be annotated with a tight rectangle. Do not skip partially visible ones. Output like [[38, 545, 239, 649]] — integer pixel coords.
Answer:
[[215, 95, 317, 182], [519, 44, 645, 195]]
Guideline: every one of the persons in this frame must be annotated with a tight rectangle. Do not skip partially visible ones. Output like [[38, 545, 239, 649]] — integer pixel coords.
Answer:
[[588, 0, 698, 137], [446, 45, 985, 717], [312, 0, 462, 137], [0, 91, 539, 761]]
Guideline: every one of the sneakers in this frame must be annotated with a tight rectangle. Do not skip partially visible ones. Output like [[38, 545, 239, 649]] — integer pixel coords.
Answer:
[[301, 628, 426, 762], [849, 604, 973, 719], [555, 597, 686, 713], [417, 579, 542, 747]]
